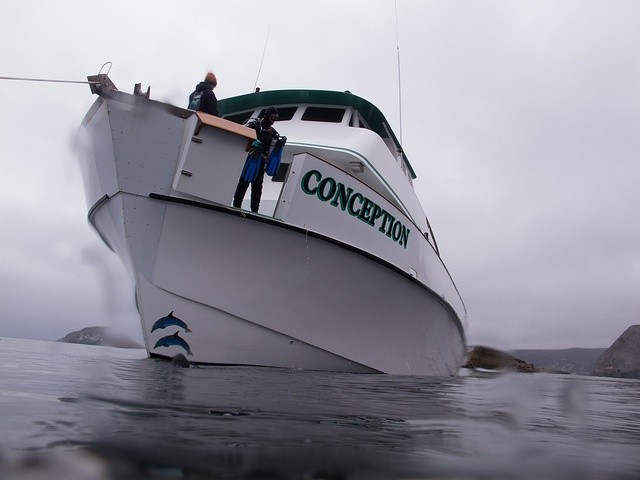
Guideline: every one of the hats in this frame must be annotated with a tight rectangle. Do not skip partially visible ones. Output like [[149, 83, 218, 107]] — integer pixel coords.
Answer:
[[205, 72, 217, 87]]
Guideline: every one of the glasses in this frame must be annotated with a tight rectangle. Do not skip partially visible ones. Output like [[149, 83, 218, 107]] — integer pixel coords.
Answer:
[[271, 115, 279, 122]]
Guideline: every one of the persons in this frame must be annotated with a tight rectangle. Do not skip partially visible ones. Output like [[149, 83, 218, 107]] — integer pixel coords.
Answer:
[[233, 105, 279, 212], [188, 72, 218, 117]]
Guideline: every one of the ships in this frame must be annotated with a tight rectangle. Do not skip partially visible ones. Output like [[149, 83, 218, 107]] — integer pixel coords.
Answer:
[[73, 62, 467, 377]]
